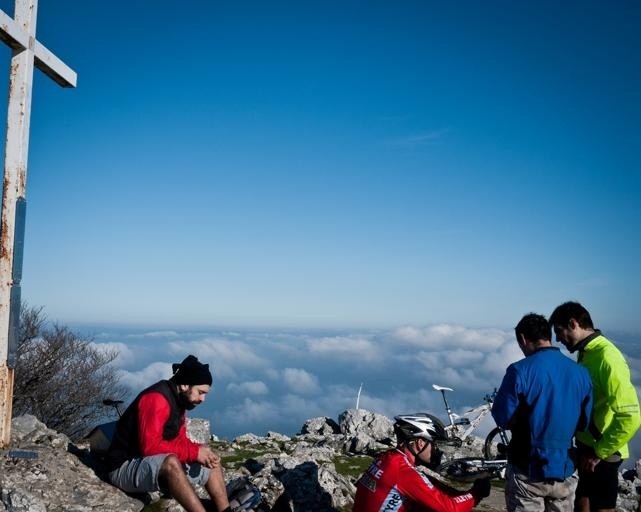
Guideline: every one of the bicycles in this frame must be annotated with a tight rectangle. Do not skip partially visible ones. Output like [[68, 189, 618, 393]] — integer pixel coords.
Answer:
[[431, 380, 511, 483]]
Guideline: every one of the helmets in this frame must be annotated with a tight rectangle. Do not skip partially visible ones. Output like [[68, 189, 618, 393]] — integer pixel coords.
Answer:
[[394, 413, 449, 442]]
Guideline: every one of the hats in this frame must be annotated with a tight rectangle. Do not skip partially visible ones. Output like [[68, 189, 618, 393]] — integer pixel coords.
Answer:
[[170, 355, 213, 385]]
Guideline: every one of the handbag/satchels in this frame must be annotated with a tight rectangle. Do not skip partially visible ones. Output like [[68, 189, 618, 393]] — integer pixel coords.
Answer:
[[226, 477, 260, 512]]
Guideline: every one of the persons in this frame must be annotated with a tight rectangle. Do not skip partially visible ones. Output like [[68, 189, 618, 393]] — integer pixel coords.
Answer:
[[106, 355, 232, 512], [352, 413, 491, 512], [491, 302, 641, 512]]
